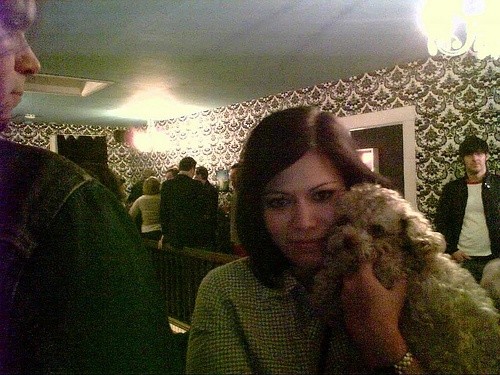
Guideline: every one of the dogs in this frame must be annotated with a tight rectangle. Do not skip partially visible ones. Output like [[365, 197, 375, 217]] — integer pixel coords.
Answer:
[[310, 180, 500, 375]]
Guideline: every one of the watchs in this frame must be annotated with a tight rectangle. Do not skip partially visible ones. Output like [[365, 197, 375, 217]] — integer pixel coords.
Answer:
[[369, 348, 415, 375]]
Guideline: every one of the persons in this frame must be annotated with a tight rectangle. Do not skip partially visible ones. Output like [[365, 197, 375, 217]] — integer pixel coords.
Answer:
[[185, 106, 428, 375], [78, 156, 249, 258], [433, 136, 500, 285], [0, 0, 184, 375]]
